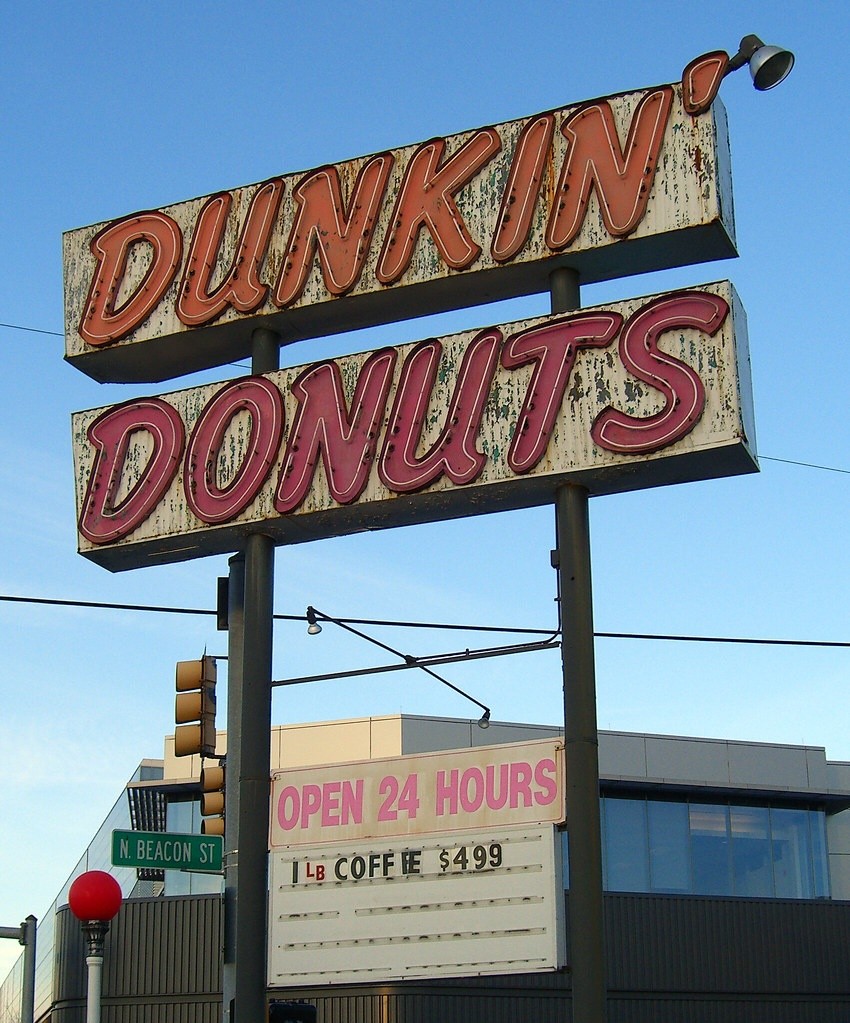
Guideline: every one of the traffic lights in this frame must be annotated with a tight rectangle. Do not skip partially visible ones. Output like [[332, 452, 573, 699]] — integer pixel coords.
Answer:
[[200, 753, 226, 836], [175, 655, 217, 757]]
